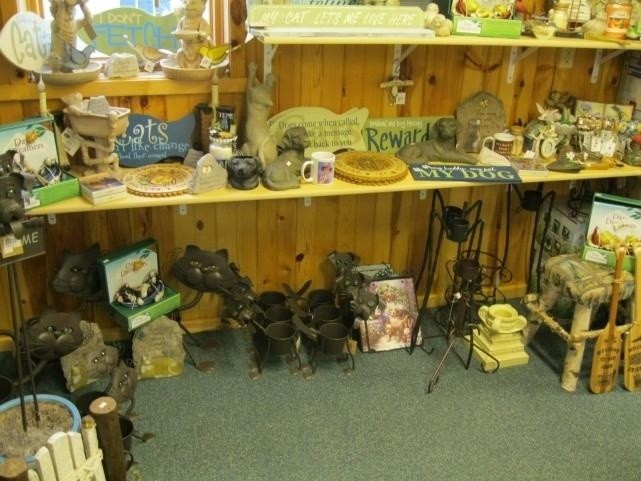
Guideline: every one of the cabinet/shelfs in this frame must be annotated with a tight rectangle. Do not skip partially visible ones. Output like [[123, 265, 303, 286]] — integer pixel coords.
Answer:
[[0, 21, 641, 349]]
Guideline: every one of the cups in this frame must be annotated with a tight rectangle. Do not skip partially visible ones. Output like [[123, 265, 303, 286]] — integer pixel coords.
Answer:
[[300, 151, 337, 188], [482, 133, 515, 160]]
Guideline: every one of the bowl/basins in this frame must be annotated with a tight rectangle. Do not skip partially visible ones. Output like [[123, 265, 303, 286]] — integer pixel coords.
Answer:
[[531, 25, 556, 41]]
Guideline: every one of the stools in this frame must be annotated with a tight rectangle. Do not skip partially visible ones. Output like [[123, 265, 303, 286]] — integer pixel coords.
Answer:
[[523, 251, 635, 392]]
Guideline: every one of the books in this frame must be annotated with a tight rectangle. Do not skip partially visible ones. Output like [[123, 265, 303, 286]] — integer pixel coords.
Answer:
[[349, 265, 424, 354], [80, 178, 127, 205]]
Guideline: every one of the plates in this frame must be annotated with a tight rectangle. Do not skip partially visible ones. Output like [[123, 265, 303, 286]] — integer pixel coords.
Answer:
[[160, 57, 227, 80], [33, 60, 104, 87]]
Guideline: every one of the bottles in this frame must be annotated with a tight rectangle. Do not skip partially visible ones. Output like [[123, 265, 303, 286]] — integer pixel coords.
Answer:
[[509, 126, 525, 157], [552, 1, 571, 33], [604, 0, 634, 40], [514, 0, 529, 33]]
